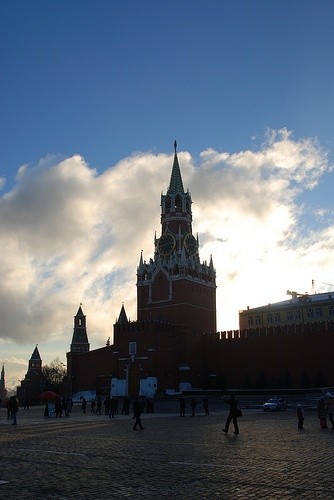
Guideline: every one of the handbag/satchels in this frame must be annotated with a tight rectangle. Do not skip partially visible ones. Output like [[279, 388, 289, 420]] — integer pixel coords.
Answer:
[[236, 409, 242, 417]]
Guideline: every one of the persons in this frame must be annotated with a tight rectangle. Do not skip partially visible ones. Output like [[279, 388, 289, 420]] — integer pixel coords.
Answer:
[[80, 396, 87, 413], [297, 404, 304, 429], [22, 399, 30, 409], [133, 396, 145, 430], [46, 395, 73, 418], [318, 393, 334, 430], [179, 395, 210, 417], [6, 397, 18, 425], [222, 393, 239, 434], [90, 396, 130, 418], [146, 398, 154, 413]]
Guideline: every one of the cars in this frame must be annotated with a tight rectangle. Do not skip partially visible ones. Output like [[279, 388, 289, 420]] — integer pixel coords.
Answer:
[[262, 397, 287, 411]]
